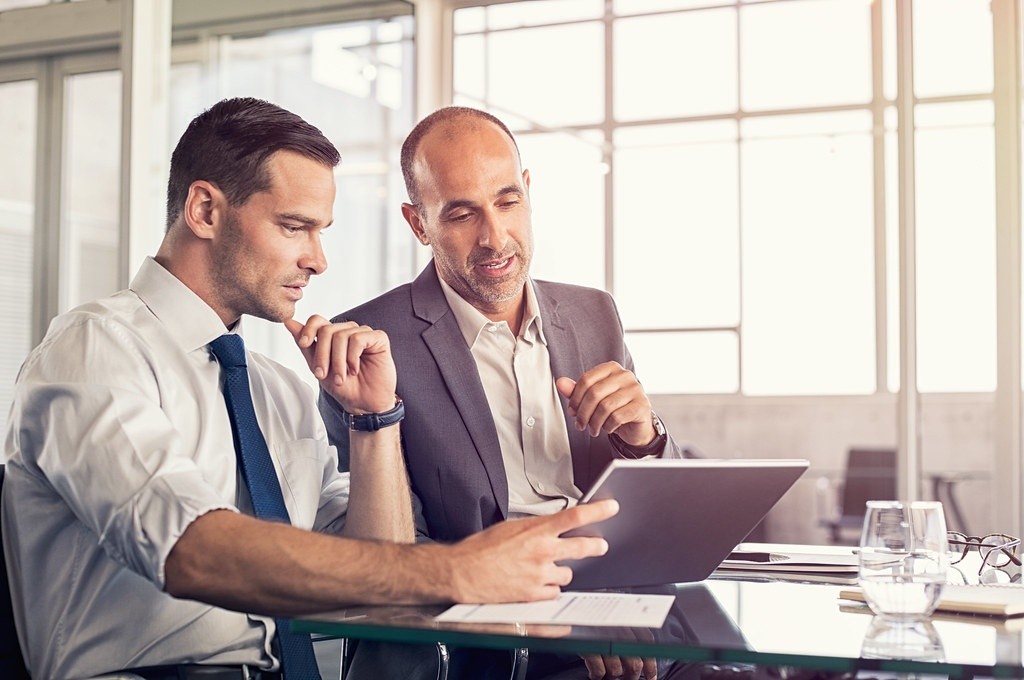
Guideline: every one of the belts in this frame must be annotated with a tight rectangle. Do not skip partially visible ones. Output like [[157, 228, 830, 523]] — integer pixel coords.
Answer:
[[120, 664, 284, 680]]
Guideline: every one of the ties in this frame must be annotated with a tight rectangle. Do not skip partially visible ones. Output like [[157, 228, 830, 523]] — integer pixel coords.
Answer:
[[212, 333, 322, 680]]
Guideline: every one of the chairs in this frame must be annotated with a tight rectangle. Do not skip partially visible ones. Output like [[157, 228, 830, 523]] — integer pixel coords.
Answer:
[[0, 464, 450, 680], [339, 635, 531, 680], [818, 448, 900, 544]]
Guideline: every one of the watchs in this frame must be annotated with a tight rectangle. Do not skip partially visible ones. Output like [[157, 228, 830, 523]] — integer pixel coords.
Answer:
[[610, 409, 667, 455], [343, 393, 406, 432]]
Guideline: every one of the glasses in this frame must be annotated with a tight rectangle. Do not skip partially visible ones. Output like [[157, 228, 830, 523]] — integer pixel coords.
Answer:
[[947, 532, 1022, 576]]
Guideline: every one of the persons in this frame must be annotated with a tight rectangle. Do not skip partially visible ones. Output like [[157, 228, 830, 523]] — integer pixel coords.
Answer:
[[315, 105, 682, 680], [0, 97, 622, 680]]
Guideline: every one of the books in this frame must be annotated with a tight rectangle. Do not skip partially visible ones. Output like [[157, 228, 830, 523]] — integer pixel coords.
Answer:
[[705, 542, 906, 586], [838, 582, 1024, 622]]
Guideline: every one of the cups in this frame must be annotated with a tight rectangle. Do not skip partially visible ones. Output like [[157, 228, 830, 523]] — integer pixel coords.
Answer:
[[857, 500, 948, 623]]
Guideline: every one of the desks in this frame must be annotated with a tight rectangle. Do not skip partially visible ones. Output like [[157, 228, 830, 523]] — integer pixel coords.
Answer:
[[288, 535, 1022, 680]]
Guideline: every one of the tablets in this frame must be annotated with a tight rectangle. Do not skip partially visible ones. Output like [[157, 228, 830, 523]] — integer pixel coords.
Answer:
[[556, 459, 811, 592]]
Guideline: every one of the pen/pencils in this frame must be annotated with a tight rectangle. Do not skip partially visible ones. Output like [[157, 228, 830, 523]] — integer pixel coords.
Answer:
[[726, 552, 791, 563]]
